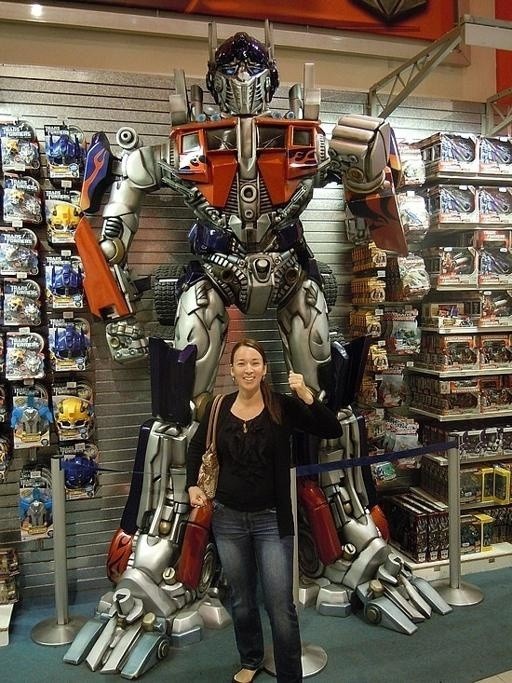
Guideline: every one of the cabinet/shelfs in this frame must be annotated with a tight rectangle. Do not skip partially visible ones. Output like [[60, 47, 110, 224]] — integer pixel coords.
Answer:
[[413, 134, 511, 553]]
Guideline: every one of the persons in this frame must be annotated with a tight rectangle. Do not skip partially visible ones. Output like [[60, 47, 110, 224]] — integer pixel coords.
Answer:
[[184, 336, 343, 683]]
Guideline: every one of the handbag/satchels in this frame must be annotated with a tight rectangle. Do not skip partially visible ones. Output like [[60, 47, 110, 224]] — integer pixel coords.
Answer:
[[196, 444, 220, 500]]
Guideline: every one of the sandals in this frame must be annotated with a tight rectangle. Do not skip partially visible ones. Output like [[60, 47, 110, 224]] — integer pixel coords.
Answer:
[[231, 663, 264, 683]]
